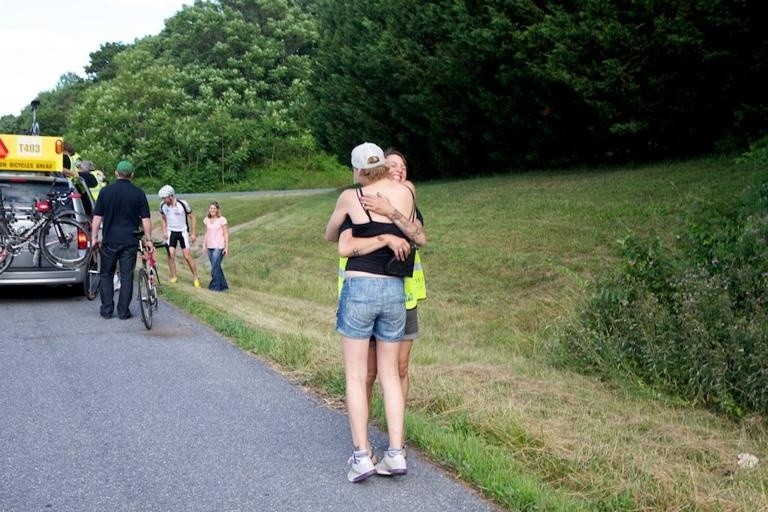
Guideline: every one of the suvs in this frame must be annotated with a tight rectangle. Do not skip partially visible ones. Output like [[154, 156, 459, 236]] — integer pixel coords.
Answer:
[[0, 134, 103, 292]]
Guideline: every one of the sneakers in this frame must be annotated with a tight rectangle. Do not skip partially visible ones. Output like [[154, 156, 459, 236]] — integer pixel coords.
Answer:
[[345, 448, 378, 483], [375, 445, 408, 476], [193, 277, 202, 289], [169, 277, 178, 283]]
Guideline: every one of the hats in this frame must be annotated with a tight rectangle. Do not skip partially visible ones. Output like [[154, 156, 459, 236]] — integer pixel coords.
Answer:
[[350, 142, 386, 169], [116, 160, 134, 178]]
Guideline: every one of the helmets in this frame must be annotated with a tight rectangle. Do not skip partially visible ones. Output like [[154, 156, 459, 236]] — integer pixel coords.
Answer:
[[158, 184, 175, 198], [90, 170, 105, 182], [78, 161, 95, 172]]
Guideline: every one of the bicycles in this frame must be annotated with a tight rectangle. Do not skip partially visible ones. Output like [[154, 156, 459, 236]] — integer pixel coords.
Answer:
[[131, 229, 161, 329], [0, 179, 96, 273]]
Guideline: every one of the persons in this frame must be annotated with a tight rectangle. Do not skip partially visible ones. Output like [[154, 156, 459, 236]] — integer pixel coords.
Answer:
[[64, 157, 122, 291], [157, 185, 201, 288], [91, 160, 156, 318], [337, 149, 428, 464], [201, 200, 229, 291], [324, 144, 419, 481], [64, 143, 106, 187]]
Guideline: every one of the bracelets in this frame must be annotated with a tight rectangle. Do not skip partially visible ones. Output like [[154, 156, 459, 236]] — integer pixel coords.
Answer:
[[146, 239, 153, 242]]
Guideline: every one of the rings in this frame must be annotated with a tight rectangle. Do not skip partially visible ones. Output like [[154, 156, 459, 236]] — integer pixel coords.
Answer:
[[399, 248, 402, 252]]
